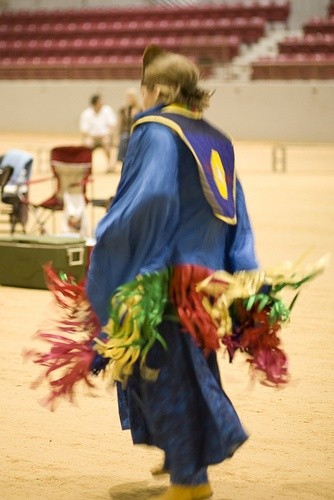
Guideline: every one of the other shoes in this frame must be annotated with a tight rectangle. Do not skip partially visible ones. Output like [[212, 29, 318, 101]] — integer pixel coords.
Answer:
[[152, 466, 168, 476], [146, 484, 212, 500]]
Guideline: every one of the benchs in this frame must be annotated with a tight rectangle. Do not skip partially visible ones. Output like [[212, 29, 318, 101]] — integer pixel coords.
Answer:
[[0, 238, 88, 290]]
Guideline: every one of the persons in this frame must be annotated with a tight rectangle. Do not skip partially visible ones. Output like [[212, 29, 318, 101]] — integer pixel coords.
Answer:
[[118, 90, 143, 162], [79, 95, 118, 174], [86, 46, 260, 500]]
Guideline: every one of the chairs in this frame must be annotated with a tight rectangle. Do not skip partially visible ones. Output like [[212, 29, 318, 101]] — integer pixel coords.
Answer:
[[0, 146, 95, 238]]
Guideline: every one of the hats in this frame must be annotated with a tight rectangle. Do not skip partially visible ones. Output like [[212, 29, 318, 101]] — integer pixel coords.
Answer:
[[140, 44, 200, 96]]
